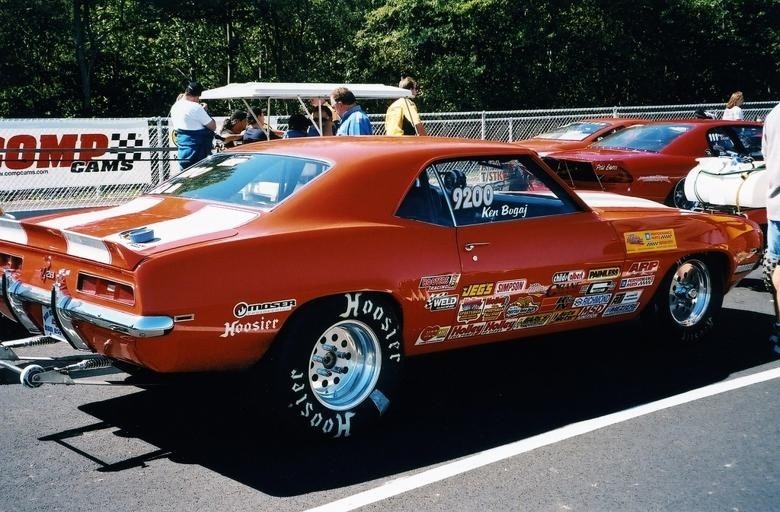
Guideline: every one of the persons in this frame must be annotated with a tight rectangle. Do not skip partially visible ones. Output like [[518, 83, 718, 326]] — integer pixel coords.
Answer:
[[722, 91, 744, 121], [760, 103, 780, 353], [170, 76, 428, 172]]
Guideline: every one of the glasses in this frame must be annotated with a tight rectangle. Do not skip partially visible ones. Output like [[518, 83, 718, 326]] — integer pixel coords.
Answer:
[[313, 118, 329, 122]]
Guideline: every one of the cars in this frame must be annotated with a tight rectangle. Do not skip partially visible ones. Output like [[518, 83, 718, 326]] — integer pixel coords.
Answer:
[[508, 117, 780, 225], [1, 133, 764, 447]]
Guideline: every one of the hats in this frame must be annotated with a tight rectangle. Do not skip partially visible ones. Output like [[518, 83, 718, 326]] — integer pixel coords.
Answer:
[[187, 82, 201, 96]]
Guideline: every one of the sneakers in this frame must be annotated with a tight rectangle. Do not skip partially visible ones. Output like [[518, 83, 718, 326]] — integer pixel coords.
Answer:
[[767, 322, 780, 354]]
